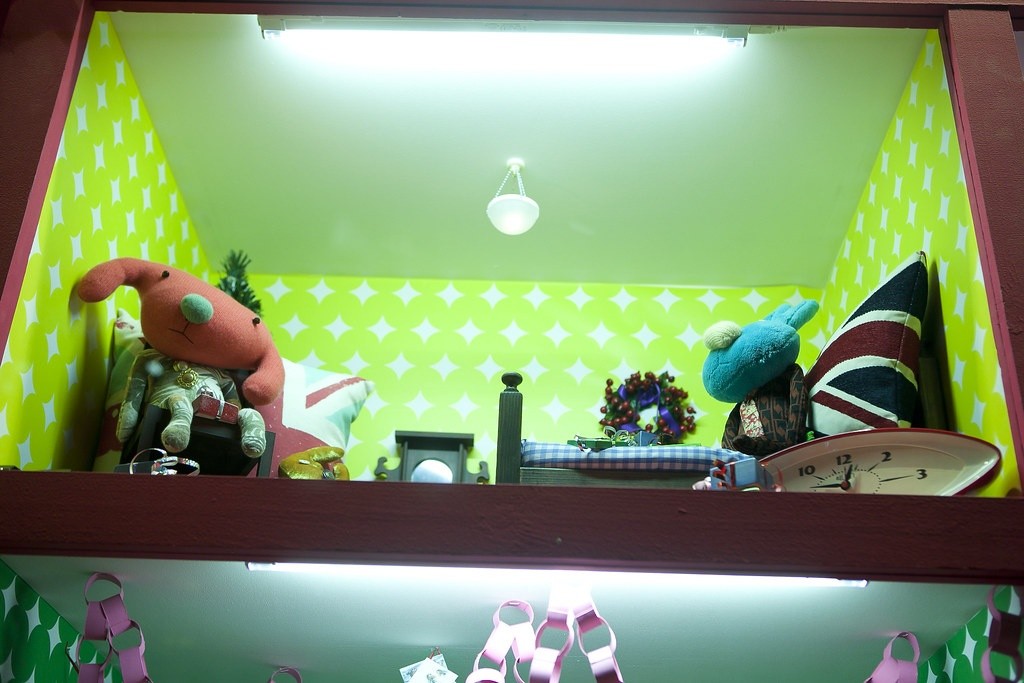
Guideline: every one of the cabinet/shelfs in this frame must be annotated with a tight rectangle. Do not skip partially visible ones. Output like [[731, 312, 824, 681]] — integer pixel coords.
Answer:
[[0, 0, 1024, 683]]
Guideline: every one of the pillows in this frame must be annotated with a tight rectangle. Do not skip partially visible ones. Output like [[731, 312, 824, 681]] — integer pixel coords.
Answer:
[[93, 309, 374, 478], [805, 249, 930, 436]]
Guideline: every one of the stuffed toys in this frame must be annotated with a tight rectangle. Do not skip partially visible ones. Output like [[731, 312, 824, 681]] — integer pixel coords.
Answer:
[[78, 257, 285, 458], [703, 298, 819, 459]]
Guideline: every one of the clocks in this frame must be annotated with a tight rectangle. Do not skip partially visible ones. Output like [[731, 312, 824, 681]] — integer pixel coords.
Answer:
[[758, 428, 1001, 497]]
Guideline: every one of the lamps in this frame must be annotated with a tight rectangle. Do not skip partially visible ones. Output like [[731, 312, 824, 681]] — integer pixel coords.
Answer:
[[258, 16, 784, 65], [244, 563, 869, 586], [487, 157, 539, 235]]
